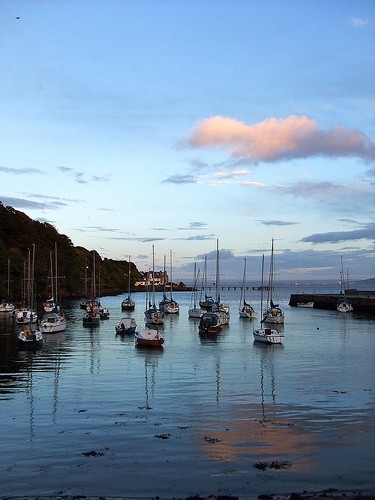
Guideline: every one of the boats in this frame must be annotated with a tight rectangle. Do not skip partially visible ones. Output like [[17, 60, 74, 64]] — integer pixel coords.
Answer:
[[189, 239, 230, 335], [14, 243, 37, 322], [253, 255, 284, 344], [159, 249, 179, 313], [144, 245, 163, 324], [10, 246, 28, 317], [18, 326, 44, 347], [40, 242, 66, 333], [80, 251, 110, 327], [115, 316, 136, 336], [44, 249, 55, 312], [135, 327, 164, 349], [263, 239, 284, 324], [238, 256, 256, 320], [121, 255, 135, 310], [1, 259, 14, 312]]
